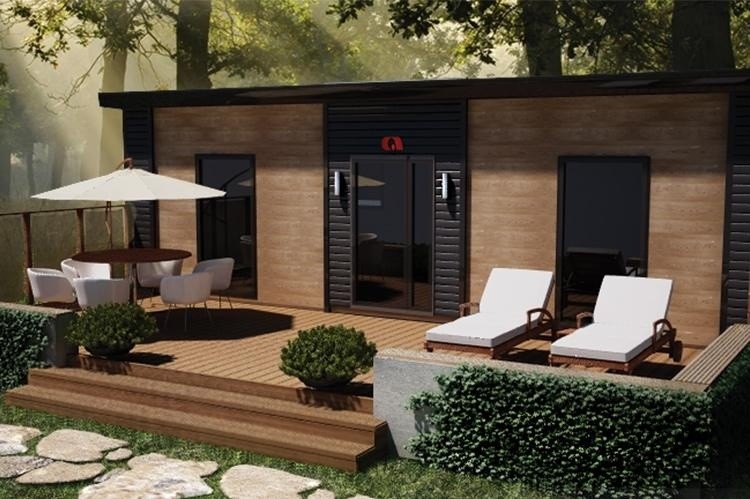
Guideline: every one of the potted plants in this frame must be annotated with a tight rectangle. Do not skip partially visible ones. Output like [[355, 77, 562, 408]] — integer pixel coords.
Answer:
[[65, 300, 160, 357], [278, 323, 377, 390]]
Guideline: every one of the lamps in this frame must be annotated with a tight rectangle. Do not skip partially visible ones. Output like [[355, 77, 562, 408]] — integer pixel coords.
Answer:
[[334, 171, 340, 195], [442, 174, 449, 198]]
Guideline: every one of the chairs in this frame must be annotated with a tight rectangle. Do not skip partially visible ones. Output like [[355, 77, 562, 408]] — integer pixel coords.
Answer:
[[548, 274, 676, 375], [359, 233, 385, 283], [241, 234, 252, 284], [566, 245, 647, 305], [27, 247, 234, 332], [424, 267, 559, 360]]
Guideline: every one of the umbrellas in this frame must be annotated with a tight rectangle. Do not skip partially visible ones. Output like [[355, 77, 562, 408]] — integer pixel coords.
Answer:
[[27, 162, 227, 310]]
